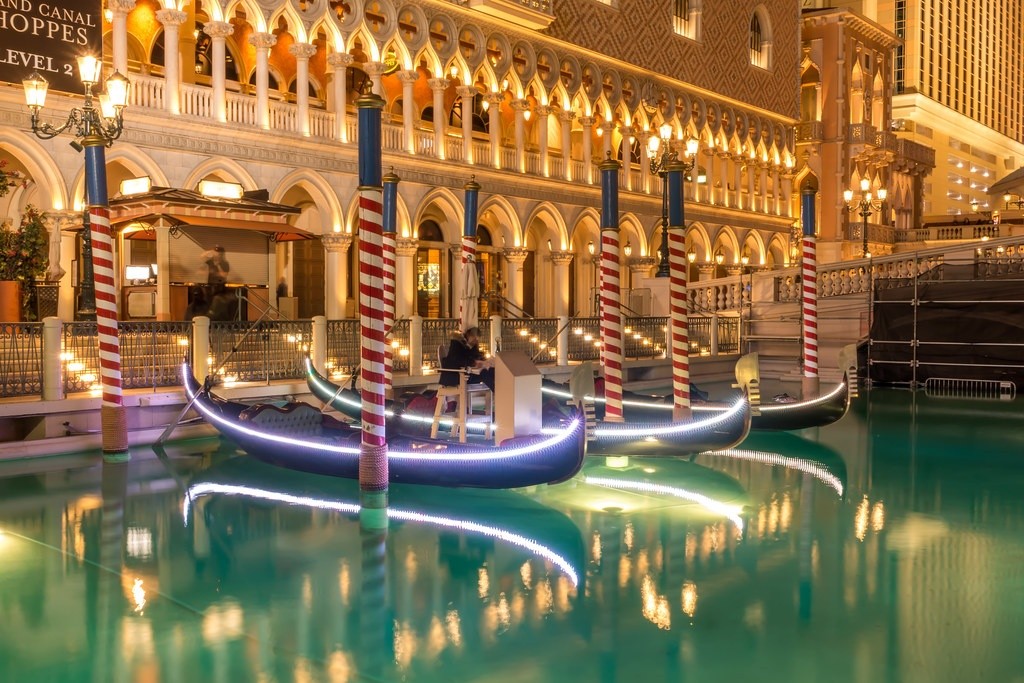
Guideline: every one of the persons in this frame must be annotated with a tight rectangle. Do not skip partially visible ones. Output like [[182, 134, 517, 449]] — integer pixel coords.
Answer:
[[436, 327, 495, 396], [200, 244, 230, 324]]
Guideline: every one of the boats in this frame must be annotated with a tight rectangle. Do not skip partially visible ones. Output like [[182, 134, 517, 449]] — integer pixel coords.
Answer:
[[180, 357, 588, 489], [542, 373, 850, 429], [305, 355, 751, 459]]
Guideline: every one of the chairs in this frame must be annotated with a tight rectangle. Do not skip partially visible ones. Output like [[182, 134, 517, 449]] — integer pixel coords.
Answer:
[[428, 345, 494, 444]]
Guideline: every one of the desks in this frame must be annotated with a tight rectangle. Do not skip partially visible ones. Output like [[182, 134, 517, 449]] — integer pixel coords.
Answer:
[[184, 282, 271, 321], [121, 284, 188, 322]]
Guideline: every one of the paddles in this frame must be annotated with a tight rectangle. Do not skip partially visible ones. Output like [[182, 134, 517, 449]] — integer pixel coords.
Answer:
[[532, 311, 580, 363], [152, 306, 272, 448], [321, 314, 405, 413]]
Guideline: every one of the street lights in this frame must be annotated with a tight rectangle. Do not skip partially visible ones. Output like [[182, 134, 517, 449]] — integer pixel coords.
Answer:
[[24, 51, 130, 335], [845, 176, 887, 274], [977, 234, 1004, 274], [646, 123, 699, 278]]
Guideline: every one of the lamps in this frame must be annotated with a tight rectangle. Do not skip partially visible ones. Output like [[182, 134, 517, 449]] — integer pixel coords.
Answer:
[[450, 65, 459, 82], [500, 76, 508, 93]]
